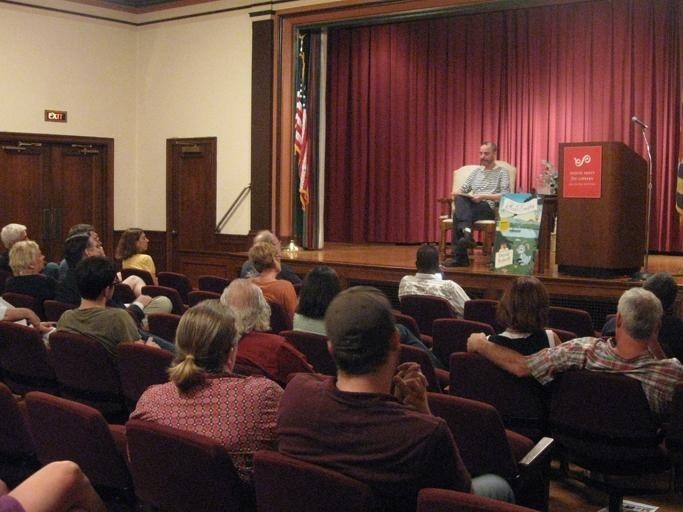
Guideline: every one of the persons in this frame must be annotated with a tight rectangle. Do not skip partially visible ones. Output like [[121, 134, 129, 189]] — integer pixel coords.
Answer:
[[1, 224, 172, 349], [443, 142, 512, 266], [129, 230, 514, 512], [0, 461, 108, 512], [467, 273, 683, 453]]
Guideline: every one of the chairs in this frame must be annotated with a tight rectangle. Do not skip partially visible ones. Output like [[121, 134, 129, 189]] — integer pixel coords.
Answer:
[[439, 160, 517, 261], [0, 259, 681, 509]]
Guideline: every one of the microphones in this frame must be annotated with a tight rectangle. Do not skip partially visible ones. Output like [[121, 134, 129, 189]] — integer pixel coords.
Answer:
[[632, 116, 648, 129]]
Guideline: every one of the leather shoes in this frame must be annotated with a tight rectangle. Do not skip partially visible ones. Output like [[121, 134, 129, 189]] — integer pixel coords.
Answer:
[[441, 255, 470, 268], [458, 237, 477, 249]]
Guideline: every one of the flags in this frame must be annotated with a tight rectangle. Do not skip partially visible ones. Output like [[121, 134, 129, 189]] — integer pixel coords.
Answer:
[[291, 49, 315, 212]]
